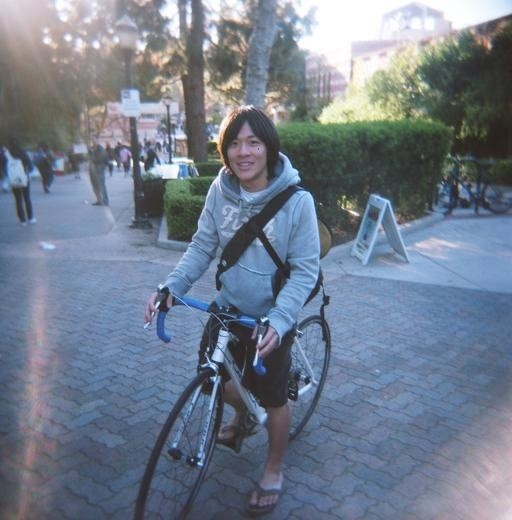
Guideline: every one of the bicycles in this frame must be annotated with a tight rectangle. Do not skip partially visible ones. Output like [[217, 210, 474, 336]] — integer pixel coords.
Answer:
[[134, 280, 331, 520], [430, 155, 511, 215]]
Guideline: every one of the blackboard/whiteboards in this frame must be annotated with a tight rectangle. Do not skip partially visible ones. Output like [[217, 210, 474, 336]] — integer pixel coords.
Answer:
[[353, 194, 387, 261]]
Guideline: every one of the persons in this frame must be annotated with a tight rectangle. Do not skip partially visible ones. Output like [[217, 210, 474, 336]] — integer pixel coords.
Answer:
[[104, 139, 169, 177], [146, 103, 321, 516], [88, 136, 110, 206], [0, 137, 57, 227], [69, 138, 84, 180]]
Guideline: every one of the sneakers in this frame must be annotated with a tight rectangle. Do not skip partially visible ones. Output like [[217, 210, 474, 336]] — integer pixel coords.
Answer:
[[92, 200, 107, 205], [20, 218, 36, 226]]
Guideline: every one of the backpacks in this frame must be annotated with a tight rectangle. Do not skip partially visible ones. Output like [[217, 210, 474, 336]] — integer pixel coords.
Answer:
[[7, 158, 27, 188]]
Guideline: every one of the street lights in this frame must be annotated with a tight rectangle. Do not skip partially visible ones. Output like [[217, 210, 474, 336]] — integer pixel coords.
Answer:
[[115, 14, 152, 229], [163, 94, 174, 163]]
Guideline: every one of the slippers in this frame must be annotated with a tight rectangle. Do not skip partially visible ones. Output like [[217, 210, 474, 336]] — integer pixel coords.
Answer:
[[243, 483, 281, 514], [218, 424, 260, 443]]
